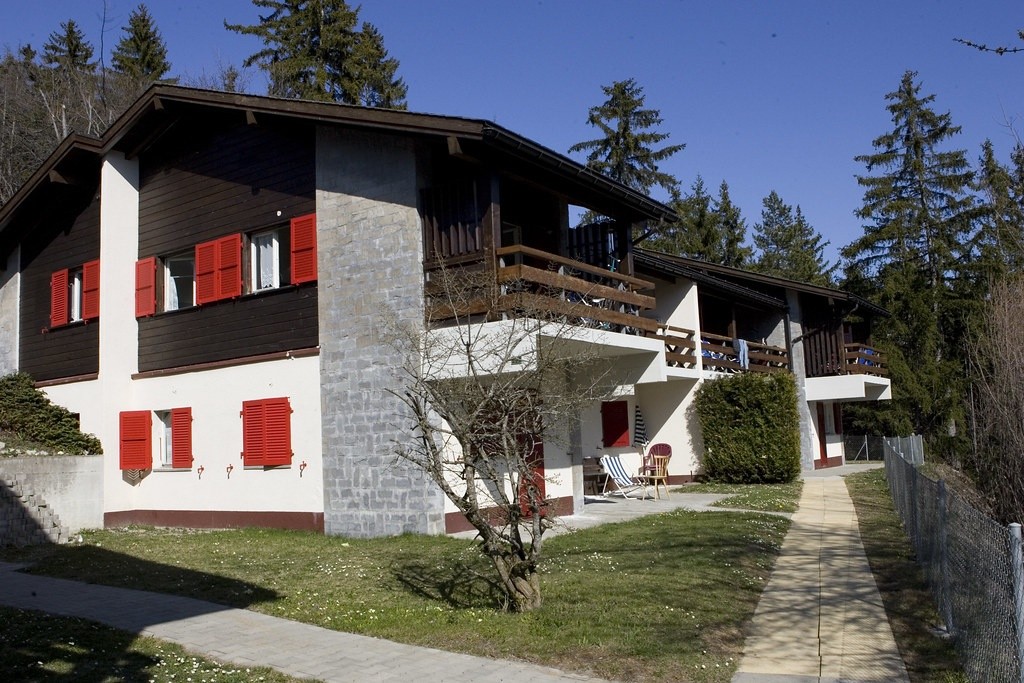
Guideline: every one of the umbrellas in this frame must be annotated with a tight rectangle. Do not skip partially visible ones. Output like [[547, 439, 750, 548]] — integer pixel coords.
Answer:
[[634, 404, 650, 475]]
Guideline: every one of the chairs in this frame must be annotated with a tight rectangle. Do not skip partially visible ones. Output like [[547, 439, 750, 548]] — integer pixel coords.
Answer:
[[634, 456, 671, 502], [600, 455, 652, 500], [643, 444, 672, 485]]
